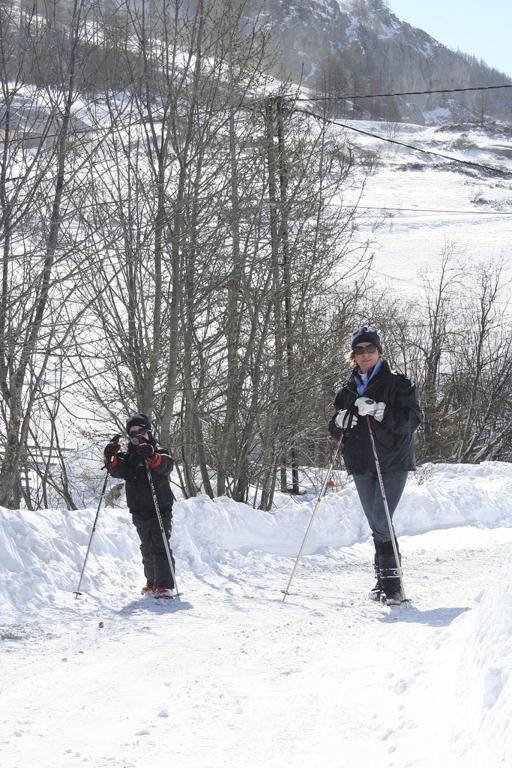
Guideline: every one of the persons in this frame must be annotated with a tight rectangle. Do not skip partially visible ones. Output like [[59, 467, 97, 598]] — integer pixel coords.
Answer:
[[102, 412, 179, 600], [326, 326, 425, 606]]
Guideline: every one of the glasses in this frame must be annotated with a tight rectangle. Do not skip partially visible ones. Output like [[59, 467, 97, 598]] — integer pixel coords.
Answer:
[[129, 427, 147, 439], [353, 344, 377, 354]]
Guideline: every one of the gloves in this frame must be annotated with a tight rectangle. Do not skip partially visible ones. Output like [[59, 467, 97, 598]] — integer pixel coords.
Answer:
[[353, 396, 386, 423], [334, 409, 359, 430], [136, 442, 155, 457], [107, 442, 121, 457]]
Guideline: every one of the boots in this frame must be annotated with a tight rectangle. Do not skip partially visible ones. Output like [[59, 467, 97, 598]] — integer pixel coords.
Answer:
[[378, 553, 402, 606], [367, 553, 385, 602]]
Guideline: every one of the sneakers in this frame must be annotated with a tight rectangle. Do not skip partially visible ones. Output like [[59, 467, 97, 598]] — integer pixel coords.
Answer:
[[153, 586, 174, 600], [141, 584, 157, 593]]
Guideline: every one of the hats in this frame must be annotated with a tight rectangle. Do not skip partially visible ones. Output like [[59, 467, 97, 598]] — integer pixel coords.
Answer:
[[126, 413, 152, 436], [351, 325, 382, 351]]
